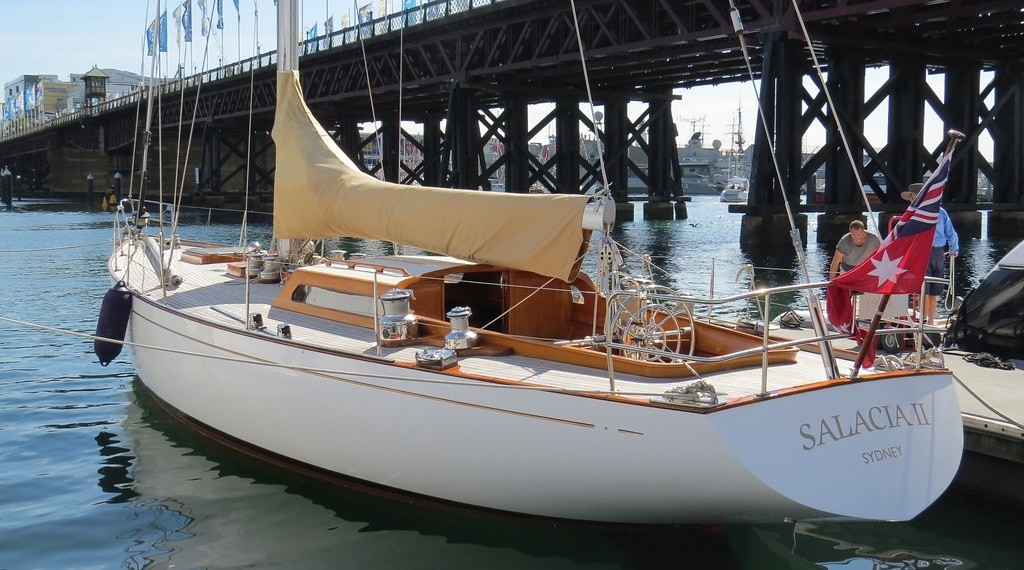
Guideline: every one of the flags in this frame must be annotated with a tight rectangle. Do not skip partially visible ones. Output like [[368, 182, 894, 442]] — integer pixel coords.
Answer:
[[0, 80, 44, 127], [827, 152, 952, 367], [147, 0, 240, 57], [308, 1, 448, 54]]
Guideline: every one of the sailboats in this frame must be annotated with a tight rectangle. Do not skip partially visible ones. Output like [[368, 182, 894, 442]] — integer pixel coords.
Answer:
[[107, 0, 963, 525]]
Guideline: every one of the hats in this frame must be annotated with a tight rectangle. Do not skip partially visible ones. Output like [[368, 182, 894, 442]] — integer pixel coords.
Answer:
[[901, 182, 924, 200]]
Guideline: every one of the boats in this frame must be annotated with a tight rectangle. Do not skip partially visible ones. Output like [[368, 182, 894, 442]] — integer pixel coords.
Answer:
[[763, 239, 1024, 465], [677, 158, 725, 194], [815, 173, 887, 204], [720, 176, 750, 203]]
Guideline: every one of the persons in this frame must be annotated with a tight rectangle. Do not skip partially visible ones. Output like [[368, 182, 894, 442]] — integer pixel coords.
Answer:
[[901, 183, 960, 325], [829, 220, 880, 281]]
